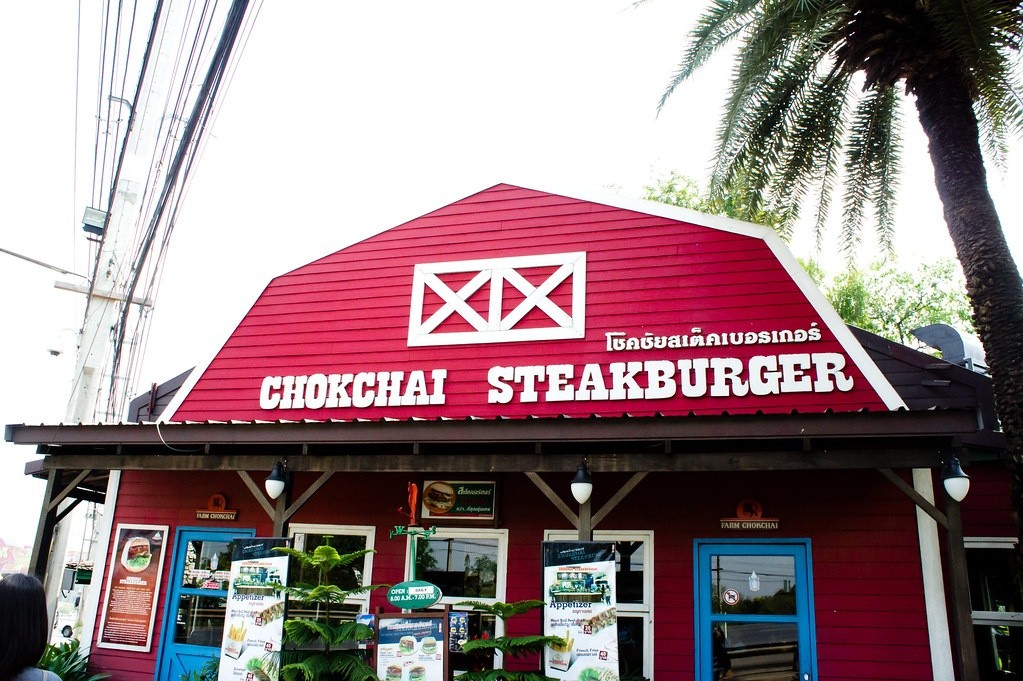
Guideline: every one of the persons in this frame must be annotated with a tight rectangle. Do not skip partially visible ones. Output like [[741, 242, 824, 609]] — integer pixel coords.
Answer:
[[0, 573, 62, 681], [595, 582, 605, 600], [604, 585, 611, 605], [550, 584, 560, 597]]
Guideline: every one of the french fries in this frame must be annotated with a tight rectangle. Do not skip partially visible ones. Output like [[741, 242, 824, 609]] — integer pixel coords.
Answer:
[[229, 621, 247, 641], [550, 631, 574, 652]]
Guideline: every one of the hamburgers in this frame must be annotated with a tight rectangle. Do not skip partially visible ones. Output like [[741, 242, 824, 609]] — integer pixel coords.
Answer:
[[127, 538, 151, 572], [421, 637, 437, 654], [399, 636, 417, 655], [408, 665, 426, 681], [385, 664, 402, 681]]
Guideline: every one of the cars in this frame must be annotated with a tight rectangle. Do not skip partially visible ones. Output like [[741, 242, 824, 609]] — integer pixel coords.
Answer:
[[54, 595, 81, 638]]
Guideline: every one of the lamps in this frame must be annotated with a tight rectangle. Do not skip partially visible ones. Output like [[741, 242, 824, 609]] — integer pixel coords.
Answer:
[[265, 459, 288, 499], [938, 446, 970, 502], [570, 462, 593, 504], [83, 206, 107, 235]]
